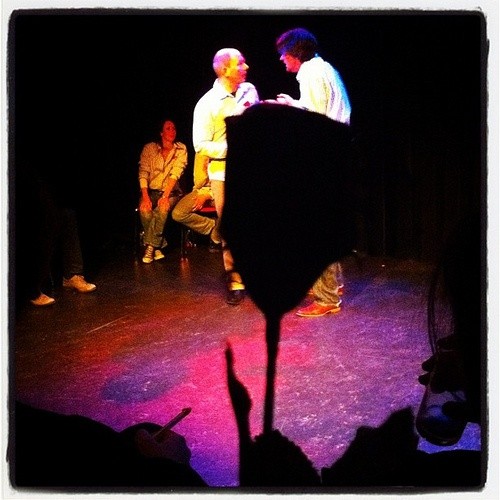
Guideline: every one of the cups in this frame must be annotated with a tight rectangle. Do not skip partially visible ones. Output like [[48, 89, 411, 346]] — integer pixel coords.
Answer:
[[416, 347, 470, 448]]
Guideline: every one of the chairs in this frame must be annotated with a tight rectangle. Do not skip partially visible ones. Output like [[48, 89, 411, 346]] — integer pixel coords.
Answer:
[[132, 201, 193, 259]]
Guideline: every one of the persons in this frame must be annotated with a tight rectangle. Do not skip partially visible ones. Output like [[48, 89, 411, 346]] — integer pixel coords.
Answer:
[[139, 118, 188, 263], [172, 48, 283, 305], [418, 335, 482, 424], [261, 27, 351, 317], [14, 399, 211, 486], [29, 179, 97, 307]]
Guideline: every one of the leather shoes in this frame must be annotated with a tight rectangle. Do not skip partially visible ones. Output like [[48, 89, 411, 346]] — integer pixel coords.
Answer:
[[226, 290, 247, 306], [208, 238, 222, 254]]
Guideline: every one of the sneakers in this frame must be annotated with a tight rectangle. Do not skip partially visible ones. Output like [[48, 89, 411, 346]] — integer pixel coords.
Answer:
[[142, 247, 154, 264], [62, 274, 97, 294], [153, 249, 165, 261], [25, 290, 56, 308]]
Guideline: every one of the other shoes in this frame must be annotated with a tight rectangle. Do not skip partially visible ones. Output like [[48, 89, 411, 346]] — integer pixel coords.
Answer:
[[296, 300, 342, 317], [303, 287, 345, 299]]
